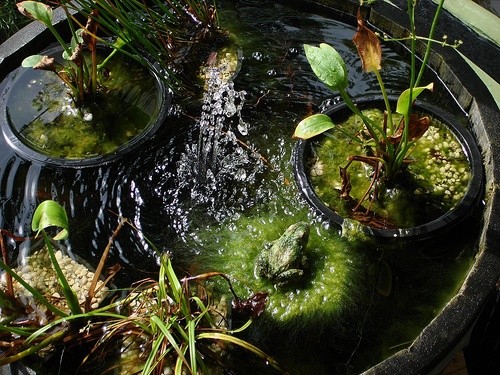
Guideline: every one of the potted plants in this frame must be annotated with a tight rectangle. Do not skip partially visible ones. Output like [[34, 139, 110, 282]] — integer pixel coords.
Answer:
[[60, 0, 248, 100], [292, 0, 485, 242], [0, 1, 172, 168], [0, 201, 120, 364], [76, 236, 284, 375]]
